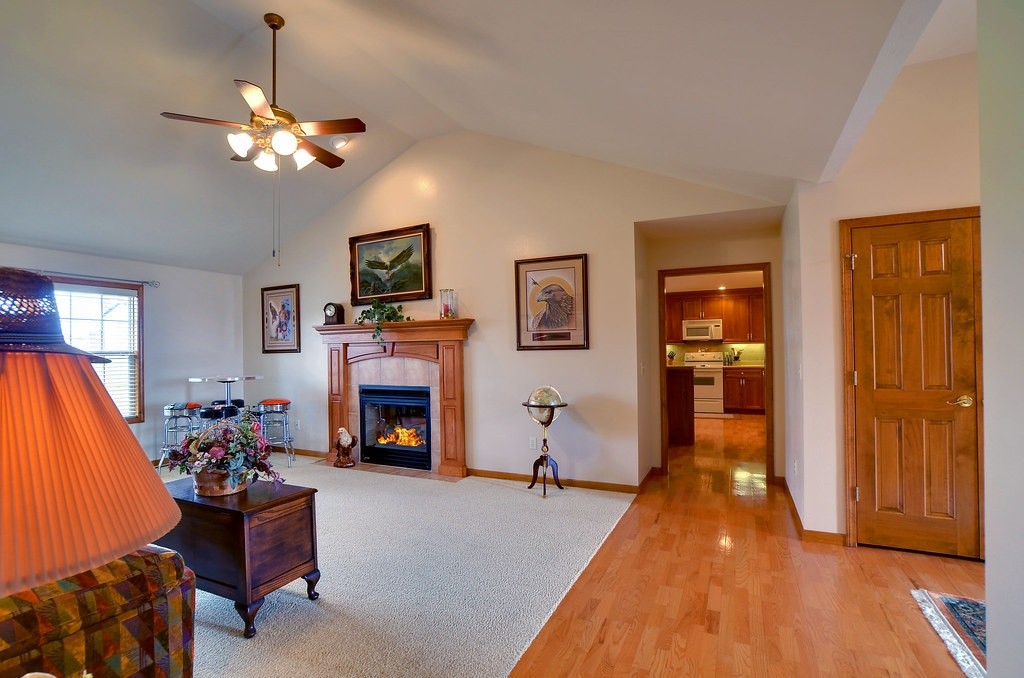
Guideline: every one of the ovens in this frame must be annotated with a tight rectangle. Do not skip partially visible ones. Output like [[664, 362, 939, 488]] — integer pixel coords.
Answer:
[[694, 369, 724, 413]]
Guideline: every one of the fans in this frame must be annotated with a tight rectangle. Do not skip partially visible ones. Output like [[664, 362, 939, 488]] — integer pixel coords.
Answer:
[[161, 13, 366, 170]]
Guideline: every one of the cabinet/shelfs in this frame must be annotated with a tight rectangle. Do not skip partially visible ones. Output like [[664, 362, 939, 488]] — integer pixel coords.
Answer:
[[665, 286, 767, 343], [150, 478, 322, 638], [666, 368, 695, 447], [721, 367, 765, 416]]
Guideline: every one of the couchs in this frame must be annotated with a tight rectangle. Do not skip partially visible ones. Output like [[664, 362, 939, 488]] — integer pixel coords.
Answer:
[[0, 542, 196, 678]]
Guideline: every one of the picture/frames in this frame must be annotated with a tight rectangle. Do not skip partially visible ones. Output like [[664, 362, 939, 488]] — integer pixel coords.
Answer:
[[514, 253, 589, 351], [261, 283, 300, 354], [349, 223, 433, 306]]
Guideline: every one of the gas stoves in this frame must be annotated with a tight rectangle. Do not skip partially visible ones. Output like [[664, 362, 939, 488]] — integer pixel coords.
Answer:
[[684, 352, 724, 368]]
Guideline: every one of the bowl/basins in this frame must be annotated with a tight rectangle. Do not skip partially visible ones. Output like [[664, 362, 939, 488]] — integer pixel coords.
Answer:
[[734, 357, 740, 360]]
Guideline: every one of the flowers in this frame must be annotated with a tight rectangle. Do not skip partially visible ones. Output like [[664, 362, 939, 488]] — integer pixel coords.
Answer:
[[167, 420, 287, 490]]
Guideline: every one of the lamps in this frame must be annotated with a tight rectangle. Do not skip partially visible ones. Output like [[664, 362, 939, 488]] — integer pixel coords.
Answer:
[[0, 266, 182, 599], [228, 126, 316, 267]]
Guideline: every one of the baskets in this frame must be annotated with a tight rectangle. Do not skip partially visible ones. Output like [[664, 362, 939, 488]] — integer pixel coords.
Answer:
[[188, 422, 250, 496]]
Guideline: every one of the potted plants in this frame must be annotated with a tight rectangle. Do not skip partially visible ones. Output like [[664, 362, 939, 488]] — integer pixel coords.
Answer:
[[355, 298, 417, 351]]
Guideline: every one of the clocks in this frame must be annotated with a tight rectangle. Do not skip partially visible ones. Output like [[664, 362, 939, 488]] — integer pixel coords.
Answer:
[[324, 302, 344, 325]]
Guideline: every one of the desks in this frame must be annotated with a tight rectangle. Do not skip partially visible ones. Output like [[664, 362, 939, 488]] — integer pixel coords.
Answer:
[[188, 375, 264, 405]]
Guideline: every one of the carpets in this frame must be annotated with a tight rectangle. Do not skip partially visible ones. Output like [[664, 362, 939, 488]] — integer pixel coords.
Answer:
[[151, 450, 637, 678], [911, 589, 988, 678]]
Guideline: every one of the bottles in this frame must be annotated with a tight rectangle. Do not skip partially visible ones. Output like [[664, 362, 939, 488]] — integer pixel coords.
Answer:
[[725, 354, 732, 365], [698, 347, 709, 353], [439, 289, 454, 319]]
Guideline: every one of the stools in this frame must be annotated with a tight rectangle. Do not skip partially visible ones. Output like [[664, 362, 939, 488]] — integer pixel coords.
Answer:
[[157, 398, 244, 475], [257, 399, 295, 468]]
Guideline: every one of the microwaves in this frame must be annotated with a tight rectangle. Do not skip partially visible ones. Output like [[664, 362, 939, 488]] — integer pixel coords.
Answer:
[[682, 319, 723, 341]]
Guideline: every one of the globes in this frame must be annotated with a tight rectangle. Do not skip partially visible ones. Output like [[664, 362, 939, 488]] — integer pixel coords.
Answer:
[[522, 385, 568, 428]]
